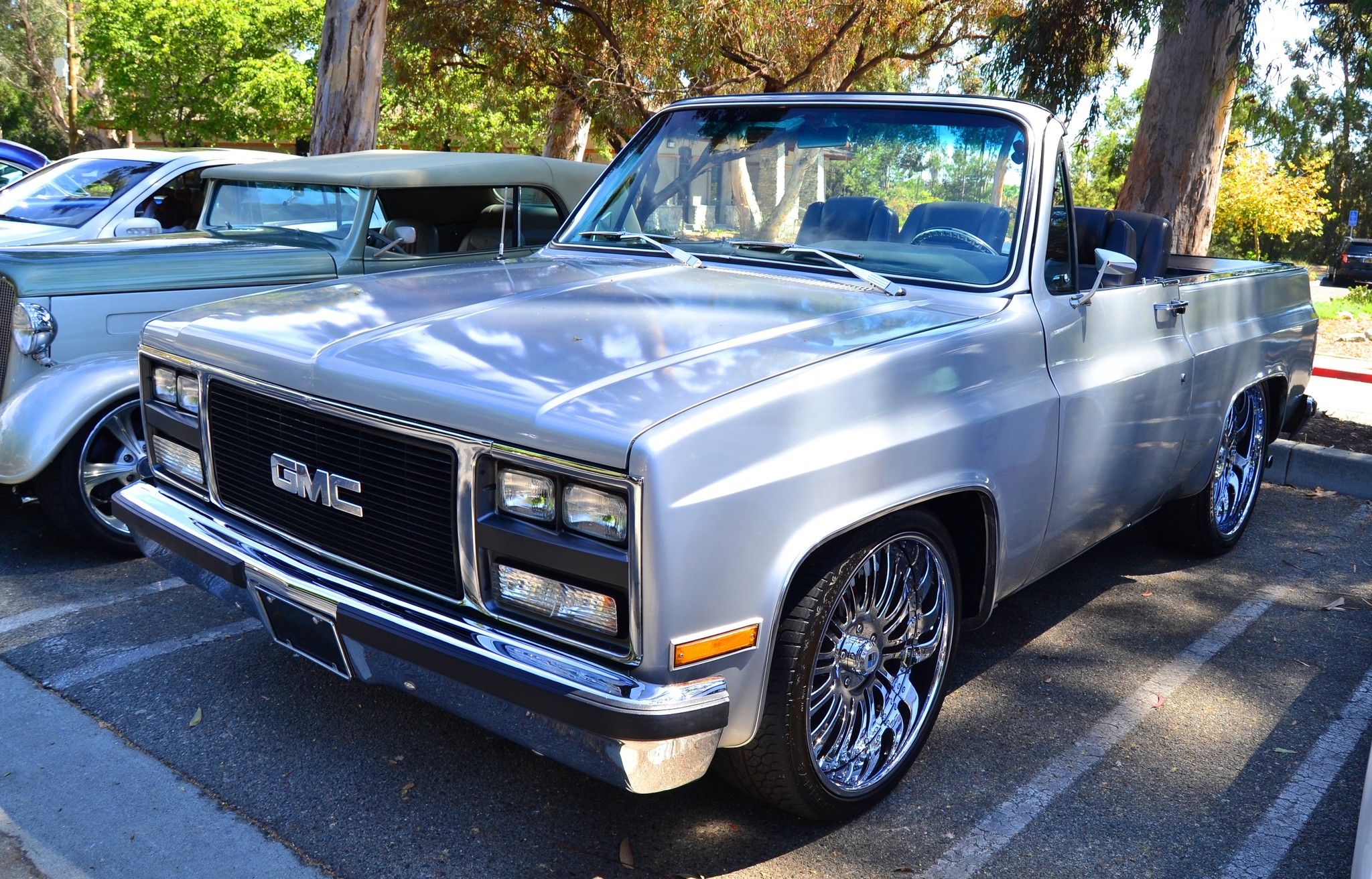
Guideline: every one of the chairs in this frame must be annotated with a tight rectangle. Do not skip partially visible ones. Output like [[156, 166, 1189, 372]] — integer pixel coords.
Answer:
[[1045, 205, 1173, 292], [898, 201, 1010, 255], [794, 195, 899, 245], [457, 204, 525, 252], [375, 219, 439, 254]]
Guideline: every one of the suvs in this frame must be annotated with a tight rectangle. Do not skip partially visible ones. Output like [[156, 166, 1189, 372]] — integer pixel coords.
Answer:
[[1326, 236, 1372, 286]]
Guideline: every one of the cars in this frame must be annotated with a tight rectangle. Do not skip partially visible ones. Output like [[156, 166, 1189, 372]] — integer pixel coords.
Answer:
[[0, 146, 390, 249], [0, 148, 647, 562], [0, 138, 90, 199]]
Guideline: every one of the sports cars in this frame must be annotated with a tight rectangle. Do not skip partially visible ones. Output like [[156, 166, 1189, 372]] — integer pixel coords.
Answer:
[[112, 92, 1319, 824]]
